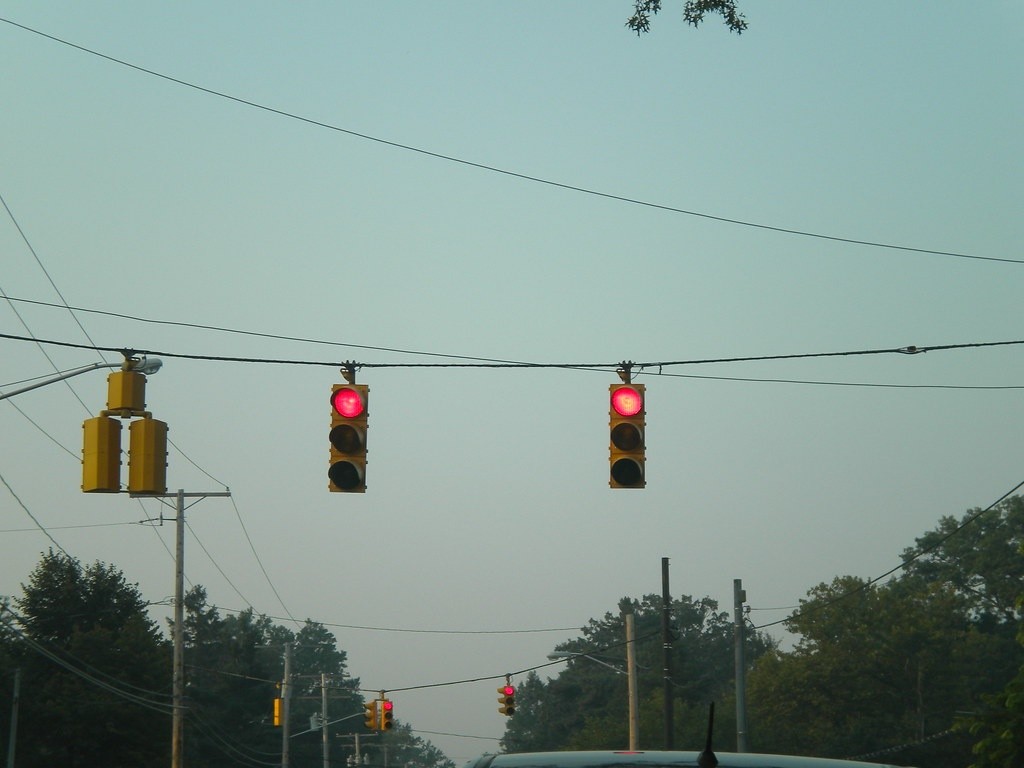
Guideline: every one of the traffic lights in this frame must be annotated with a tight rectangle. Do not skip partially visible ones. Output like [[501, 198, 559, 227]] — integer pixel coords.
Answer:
[[364, 701, 378, 729], [503, 685, 515, 716], [497, 687, 506, 715], [607, 383, 648, 490], [327, 383, 371, 494], [381, 701, 393, 731]]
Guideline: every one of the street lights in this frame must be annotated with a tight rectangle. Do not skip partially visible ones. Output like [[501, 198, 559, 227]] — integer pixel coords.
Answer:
[[547, 650, 640, 751]]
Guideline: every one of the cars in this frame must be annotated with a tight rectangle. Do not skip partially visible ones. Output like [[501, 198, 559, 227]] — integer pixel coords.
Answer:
[[462, 751, 910, 768]]
